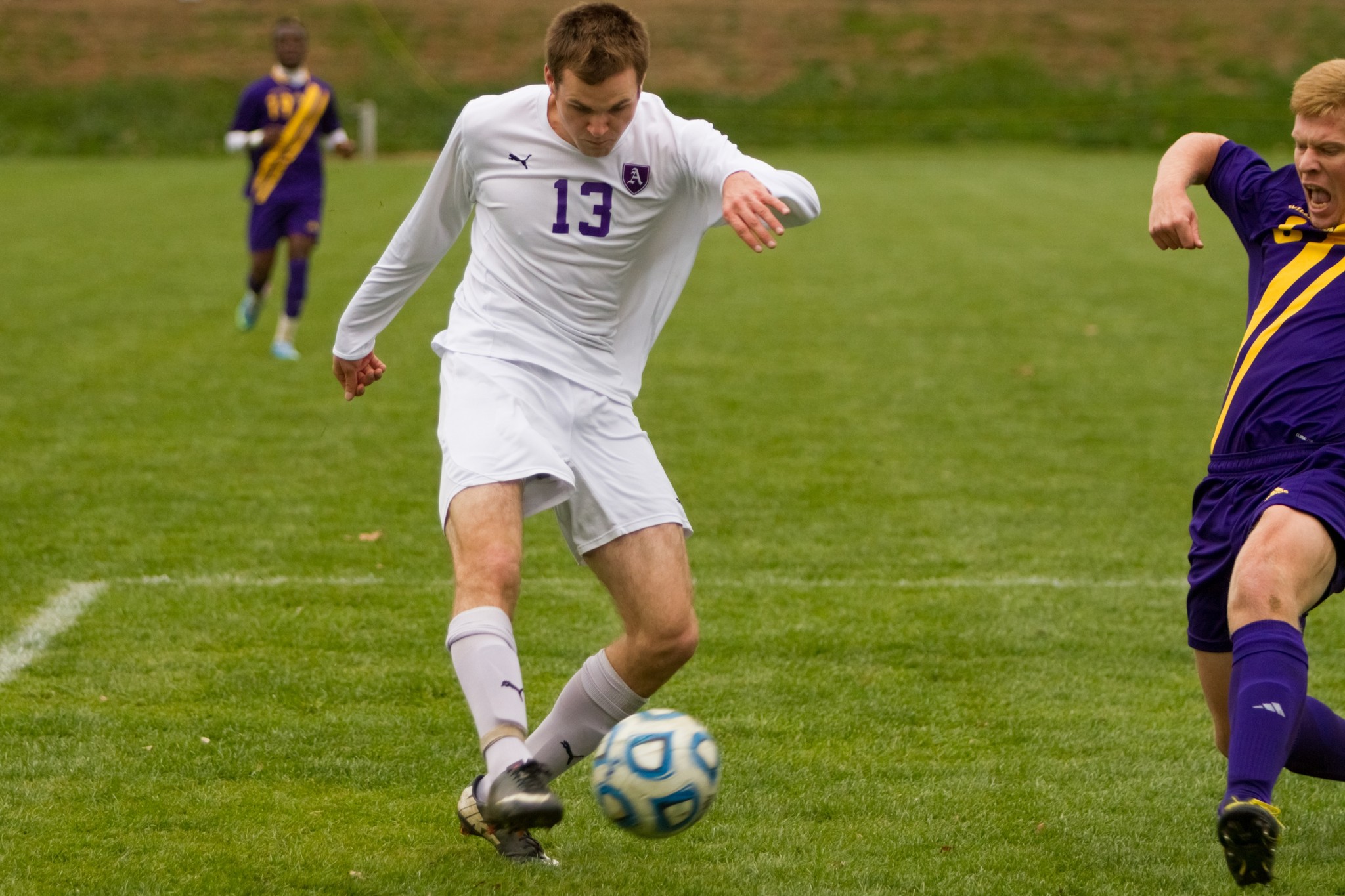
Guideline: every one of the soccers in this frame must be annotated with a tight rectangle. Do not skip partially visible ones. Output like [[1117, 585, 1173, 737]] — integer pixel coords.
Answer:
[[590, 706, 719, 840]]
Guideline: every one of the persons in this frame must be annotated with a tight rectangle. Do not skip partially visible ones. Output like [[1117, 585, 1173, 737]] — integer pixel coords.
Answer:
[[1144, 54, 1345, 887], [333, 1, 827, 870], [218, 16, 361, 361]]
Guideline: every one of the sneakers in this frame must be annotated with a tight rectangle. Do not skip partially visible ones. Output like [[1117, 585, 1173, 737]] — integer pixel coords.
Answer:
[[266, 334, 302, 363], [453, 775, 564, 870], [468, 758, 567, 829], [1214, 794, 1285, 894], [236, 282, 272, 334]]
